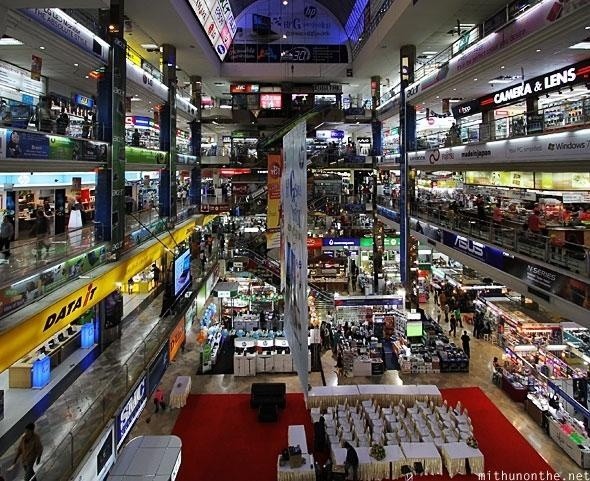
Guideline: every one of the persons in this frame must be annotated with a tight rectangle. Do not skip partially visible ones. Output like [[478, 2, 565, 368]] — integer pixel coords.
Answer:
[[56, 112, 66, 134], [480, 322, 489, 339], [390, 189, 397, 209], [42, 200, 55, 219], [449, 123, 455, 136], [492, 357, 500, 367], [313, 416, 326, 454], [343, 441, 359, 481], [72, 199, 84, 212], [30, 210, 50, 264], [527, 208, 549, 244], [12, 423, 44, 481], [132, 128, 141, 147], [448, 315, 457, 338], [363, 186, 368, 194], [208, 234, 213, 254], [351, 266, 359, 293], [148, 265, 160, 288], [320, 320, 374, 376], [453, 307, 464, 329], [473, 318, 481, 337], [348, 94, 353, 108], [475, 194, 483, 205], [138, 190, 144, 212], [80, 117, 90, 139], [444, 301, 449, 323], [128, 279, 133, 295], [437, 303, 442, 325], [328, 142, 331, 150], [199, 250, 207, 273], [476, 318, 483, 339], [455, 125, 461, 141], [37, 103, 52, 134], [391, 88, 395, 97], [220, 235, 226, 254], [460, 331, 470, 360], [144, 201, 154, 229], [332, 141, 337, 147], [127, 194, 134, 215], [491, 203, 505, 241], [0, 216, 13, 259]]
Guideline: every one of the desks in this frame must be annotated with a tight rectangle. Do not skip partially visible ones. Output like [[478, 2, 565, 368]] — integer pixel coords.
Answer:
[[170, 376, 191, 408], [329, 442, 485, 481], [307, 385, 443, 412], [277, 424, 316, 481]]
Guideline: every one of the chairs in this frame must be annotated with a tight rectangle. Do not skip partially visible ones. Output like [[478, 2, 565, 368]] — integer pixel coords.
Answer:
[[310, 396, 474, 449]]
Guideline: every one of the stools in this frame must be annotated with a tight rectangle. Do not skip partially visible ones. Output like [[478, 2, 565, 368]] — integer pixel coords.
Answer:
[[258, 405, 278, 423]]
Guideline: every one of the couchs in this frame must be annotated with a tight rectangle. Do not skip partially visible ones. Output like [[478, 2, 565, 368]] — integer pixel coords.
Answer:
[[250, 383, 286, 410]]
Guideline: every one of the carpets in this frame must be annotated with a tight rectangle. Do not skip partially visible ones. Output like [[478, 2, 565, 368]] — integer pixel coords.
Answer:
[[166, 388, 563, 481]]
